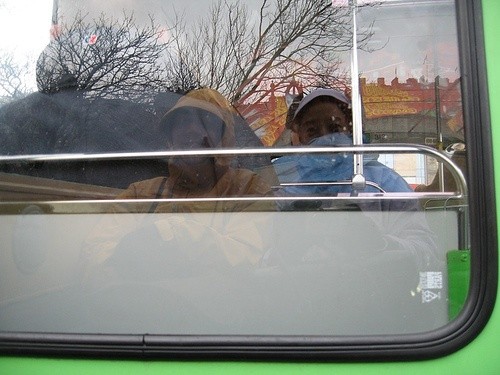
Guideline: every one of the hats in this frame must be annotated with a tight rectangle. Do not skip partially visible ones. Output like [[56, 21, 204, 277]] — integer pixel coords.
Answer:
[[292, 86, 349, 122]]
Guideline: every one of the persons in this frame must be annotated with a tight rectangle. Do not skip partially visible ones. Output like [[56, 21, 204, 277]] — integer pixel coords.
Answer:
[[73, 86, 279, 300], [271, 88, 440, 292]]
